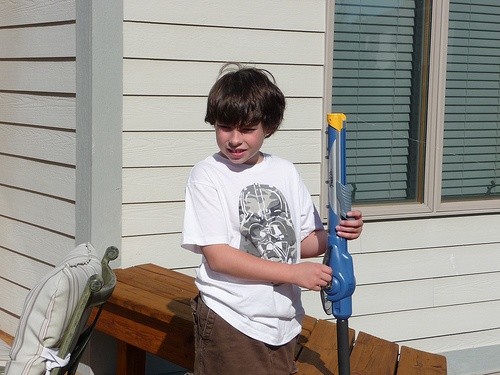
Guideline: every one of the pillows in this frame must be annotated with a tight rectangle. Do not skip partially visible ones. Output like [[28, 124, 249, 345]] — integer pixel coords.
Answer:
[[3, 241, 102, 374]]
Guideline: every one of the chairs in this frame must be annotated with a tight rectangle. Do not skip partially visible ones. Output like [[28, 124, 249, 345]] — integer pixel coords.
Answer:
[[50, 245, 120, 375]]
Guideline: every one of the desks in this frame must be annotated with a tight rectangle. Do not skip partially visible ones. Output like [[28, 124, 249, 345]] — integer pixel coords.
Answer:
[[89, 264, 448, 374]]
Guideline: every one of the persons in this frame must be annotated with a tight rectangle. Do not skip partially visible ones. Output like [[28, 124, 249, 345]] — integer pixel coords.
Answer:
[[180, 61, 362, 374]]
